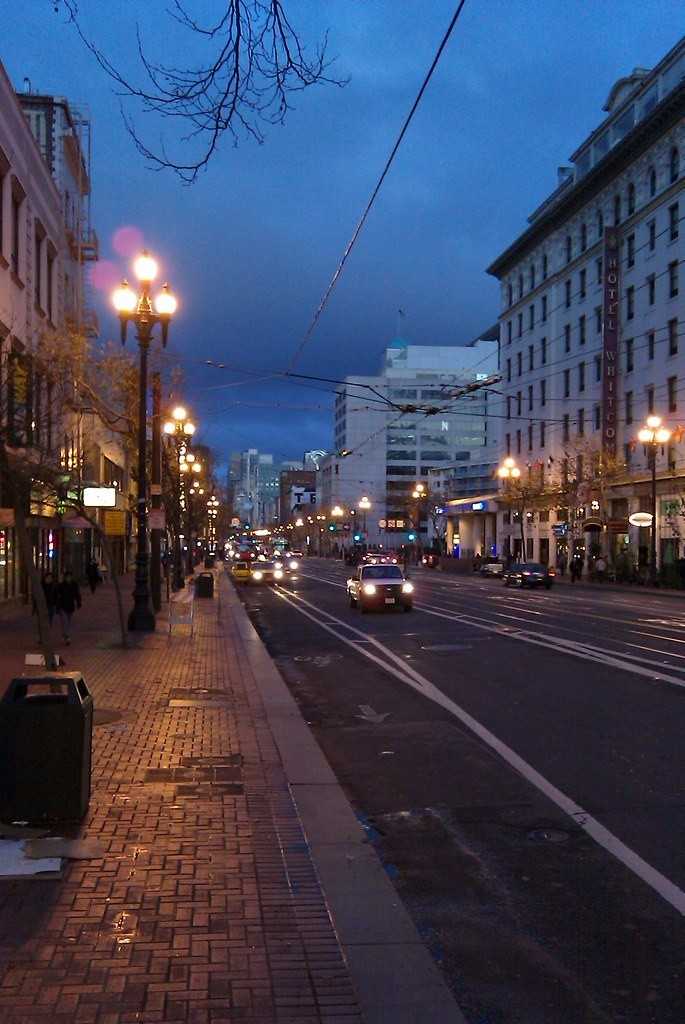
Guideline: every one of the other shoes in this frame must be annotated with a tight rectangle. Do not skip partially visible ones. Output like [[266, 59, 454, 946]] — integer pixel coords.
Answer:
[[65, 637, 71, 645]]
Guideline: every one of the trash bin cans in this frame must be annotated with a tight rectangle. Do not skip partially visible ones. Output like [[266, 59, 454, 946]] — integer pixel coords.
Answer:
[[343, 551, 352, 566], [194, 571, 214, 599], [1, 671, 94, 826]]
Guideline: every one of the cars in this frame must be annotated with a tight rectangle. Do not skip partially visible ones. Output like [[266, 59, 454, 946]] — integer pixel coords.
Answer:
[[502, 562, 555, 590], [223, 539, 305, 584], [346, 563, 414, 613]]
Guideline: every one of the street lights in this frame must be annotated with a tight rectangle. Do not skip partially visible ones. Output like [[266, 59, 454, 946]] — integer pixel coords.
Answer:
[[331, 506, 344, 560], [315, 515, 327, 558], [111, 249, 179, 634], [412, 484, 428, 560], [496, 456, 522, 564], [162, 403, 196, 590], [206, 495, 221, 553], [358, 497, 371, 555], [635, 413, 673, 588], [179, 452, 203, 574]]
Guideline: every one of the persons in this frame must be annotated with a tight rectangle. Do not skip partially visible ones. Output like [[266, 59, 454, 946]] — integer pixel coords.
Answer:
[[595, 557, 606, 583], [86, 558, 98, 593], [570, 558, 584, 583], [54, 571, 81, 647], [32, 571, 55, 645]]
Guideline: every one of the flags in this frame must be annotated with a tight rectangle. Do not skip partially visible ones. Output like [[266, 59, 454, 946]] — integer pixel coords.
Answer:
[[399, 310, 406, 318]]
